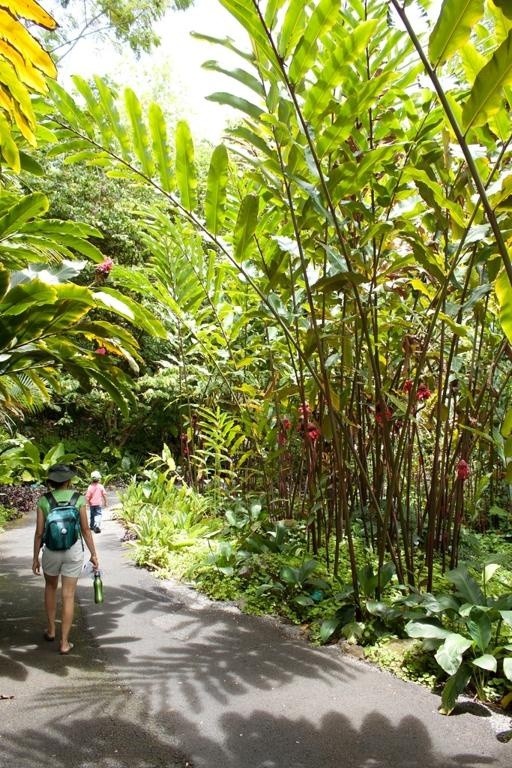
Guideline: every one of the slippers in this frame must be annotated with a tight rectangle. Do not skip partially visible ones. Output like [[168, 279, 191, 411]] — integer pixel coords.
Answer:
[[44, 629, 55, 641], [59, 641, 75, 655]]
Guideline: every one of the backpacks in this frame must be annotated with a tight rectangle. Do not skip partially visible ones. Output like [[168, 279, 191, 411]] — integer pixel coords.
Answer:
[[43, 493, 81, 551]]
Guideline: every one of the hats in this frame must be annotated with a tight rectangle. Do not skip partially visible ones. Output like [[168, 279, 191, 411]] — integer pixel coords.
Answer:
[[91, 471, 102, 481], [46, 465, 75, 484]]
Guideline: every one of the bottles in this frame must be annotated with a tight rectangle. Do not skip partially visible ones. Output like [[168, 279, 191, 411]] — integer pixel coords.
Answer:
[[92, 571, 105, 604]]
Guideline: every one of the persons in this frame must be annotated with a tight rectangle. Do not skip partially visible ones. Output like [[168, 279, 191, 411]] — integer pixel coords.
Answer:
[[31, 463, 101, 655], [84, 470, 108, 533]]
